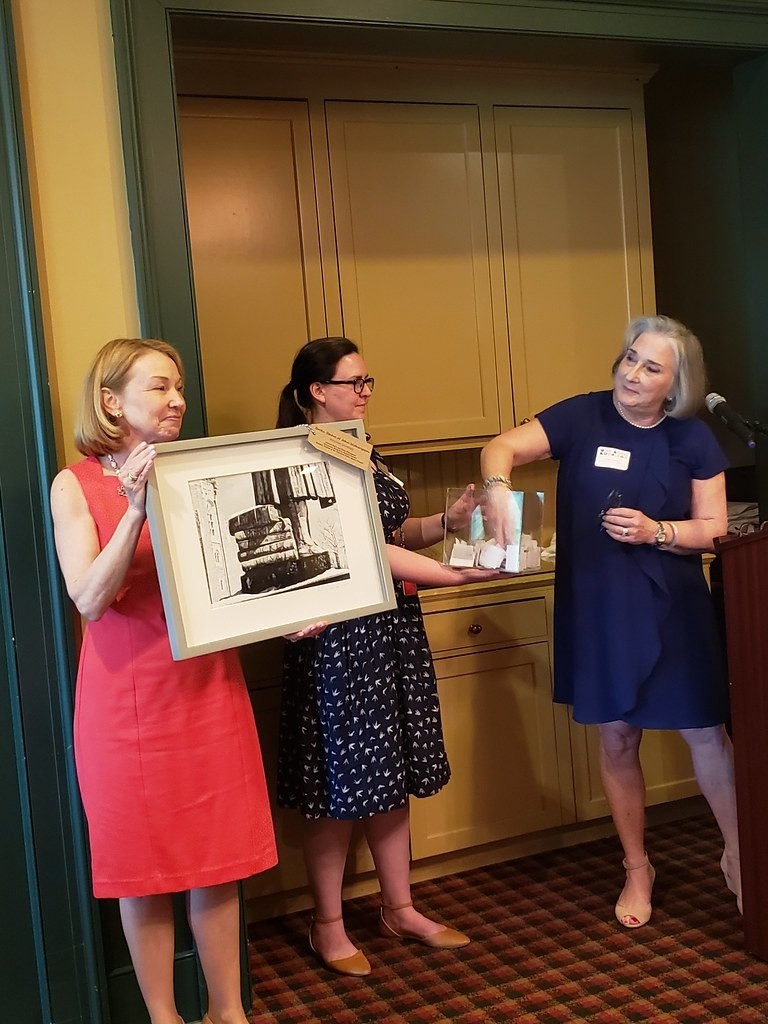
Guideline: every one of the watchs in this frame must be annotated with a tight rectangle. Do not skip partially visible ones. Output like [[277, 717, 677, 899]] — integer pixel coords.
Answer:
[[655, 521, 666, 547]]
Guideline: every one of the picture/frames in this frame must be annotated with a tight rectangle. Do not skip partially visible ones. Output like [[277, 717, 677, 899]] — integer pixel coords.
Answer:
[[144, 418, 399, 661]]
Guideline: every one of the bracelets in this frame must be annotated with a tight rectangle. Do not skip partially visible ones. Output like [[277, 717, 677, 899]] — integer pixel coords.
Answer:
[[657, 521, 678, 550], [483, 475, 512, 490], [441, 514, 455, 534]]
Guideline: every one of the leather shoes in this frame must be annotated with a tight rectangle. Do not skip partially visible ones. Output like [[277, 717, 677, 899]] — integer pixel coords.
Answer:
[[307, 911, 372, 977], [377, 899, 470, 949]]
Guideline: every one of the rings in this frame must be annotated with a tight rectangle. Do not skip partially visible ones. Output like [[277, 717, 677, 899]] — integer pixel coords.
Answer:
[[622, 528, 628, 536], [128, 473, 138, 481]]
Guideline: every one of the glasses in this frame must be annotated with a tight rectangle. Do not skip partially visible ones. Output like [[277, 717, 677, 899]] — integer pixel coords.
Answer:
[[320, 377, 374, 394]]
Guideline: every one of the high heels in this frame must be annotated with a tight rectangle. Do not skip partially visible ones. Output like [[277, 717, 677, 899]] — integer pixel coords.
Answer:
[[615, 849, 656, 928], [720, 849, 742, 916]]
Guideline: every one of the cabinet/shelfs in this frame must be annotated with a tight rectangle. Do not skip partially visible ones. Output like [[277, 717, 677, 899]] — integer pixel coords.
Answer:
[[171, 22, 329, 434], [330, 26, 657, 457], [238, 638, 380, 925], [407, 564, 712, 884]]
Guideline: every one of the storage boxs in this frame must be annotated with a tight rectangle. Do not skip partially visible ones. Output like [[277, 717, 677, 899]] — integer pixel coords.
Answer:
[[440, 487, 545, 576]]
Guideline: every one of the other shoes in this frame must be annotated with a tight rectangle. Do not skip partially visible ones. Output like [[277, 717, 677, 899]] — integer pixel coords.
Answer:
[[177, 1016, 185, 1024], [202, 1012, 213, 1024]]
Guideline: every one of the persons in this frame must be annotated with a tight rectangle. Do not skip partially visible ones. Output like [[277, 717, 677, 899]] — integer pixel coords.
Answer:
[[275, 337, 500, 976], [480, 316, 742, 927], [50, 338, 328, 1024]]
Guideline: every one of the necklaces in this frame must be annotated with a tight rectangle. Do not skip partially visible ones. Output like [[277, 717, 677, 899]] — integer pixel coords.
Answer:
[[107, 454, 127, 497]]
